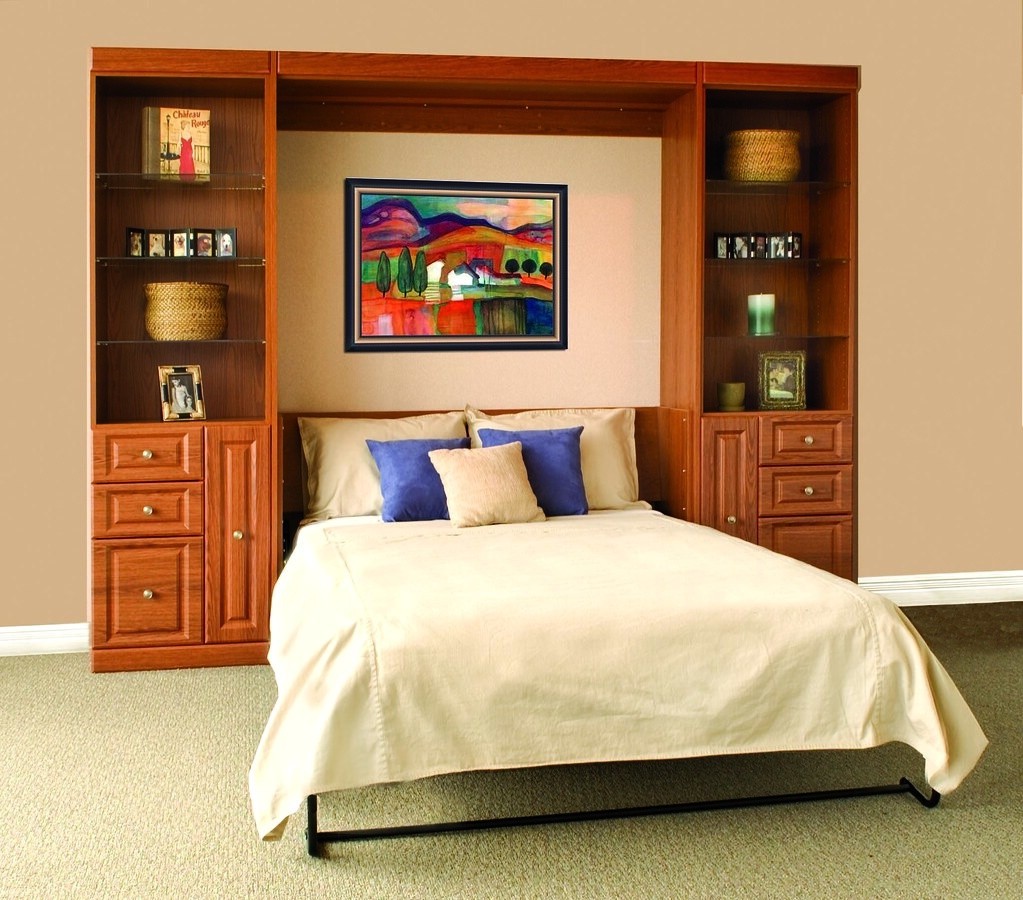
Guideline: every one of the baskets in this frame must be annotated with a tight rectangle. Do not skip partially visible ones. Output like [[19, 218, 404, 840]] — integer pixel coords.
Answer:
[[144, 282, 229, 341], [725, 129, 801, 182]]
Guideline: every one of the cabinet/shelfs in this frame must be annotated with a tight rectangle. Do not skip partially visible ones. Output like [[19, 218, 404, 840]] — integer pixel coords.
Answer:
[[84, 43, 862, 673]]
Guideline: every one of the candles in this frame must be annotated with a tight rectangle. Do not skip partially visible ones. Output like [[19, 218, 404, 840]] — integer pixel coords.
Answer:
[[748, 292, 776, 336]]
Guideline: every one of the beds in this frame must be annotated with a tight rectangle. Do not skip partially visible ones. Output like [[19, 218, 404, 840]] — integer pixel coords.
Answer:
[[246, 405, 989, 858]]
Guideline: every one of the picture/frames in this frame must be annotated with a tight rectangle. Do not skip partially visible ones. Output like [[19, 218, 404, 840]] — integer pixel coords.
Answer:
[[792, 232, 803, 259], [124, 226, 145, 257], [193, 228, 215, 258], [754, 231, 767, 259], [767, 232, 788, 259], [758, 350, 807, 411], [158, 365, 207, 422], [216, 227, 237, 258], [342, 177, 569, 353], [730, 232, 751, 259], [146, 228, 169, 257], [712, 232, 729, 259], [170, 228, 190, 257]]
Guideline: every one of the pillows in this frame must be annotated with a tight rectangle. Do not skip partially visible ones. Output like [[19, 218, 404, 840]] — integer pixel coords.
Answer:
[[464, 404, 653, 512], [366, 437, 472, 523], [295, 410, 467, 528], [425, 440, 548, 530], [477, 425, 590, 517]]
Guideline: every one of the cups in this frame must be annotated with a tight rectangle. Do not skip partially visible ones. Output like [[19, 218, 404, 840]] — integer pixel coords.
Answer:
[[718, 382, 745, 406]]
